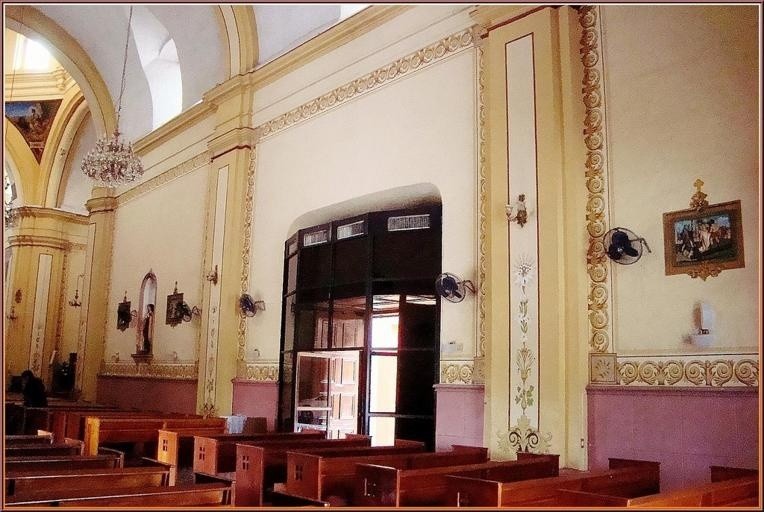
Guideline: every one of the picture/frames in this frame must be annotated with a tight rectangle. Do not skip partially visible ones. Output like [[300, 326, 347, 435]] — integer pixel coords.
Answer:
[[663, 200, 748, 279]]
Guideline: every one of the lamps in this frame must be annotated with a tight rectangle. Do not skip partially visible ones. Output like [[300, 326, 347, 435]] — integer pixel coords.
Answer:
[[80, 6, 147, 187]]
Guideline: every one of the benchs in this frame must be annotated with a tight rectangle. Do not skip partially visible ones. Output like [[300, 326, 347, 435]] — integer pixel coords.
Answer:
[[5, 395, 756, 509]]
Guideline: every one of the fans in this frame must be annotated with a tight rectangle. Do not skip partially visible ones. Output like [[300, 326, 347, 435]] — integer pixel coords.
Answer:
[[237, 293, 266, 320], [602, 226, 651, 268], [435, 272, 479, 305], [173, 299, 201, 322]]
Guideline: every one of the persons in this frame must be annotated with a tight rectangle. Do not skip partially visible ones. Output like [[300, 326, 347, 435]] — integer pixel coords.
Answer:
[[19, 368, 49, 409], [25, 108, 43, 135], [142, 303, 154, 353], [678, 220, 731, 261]]
